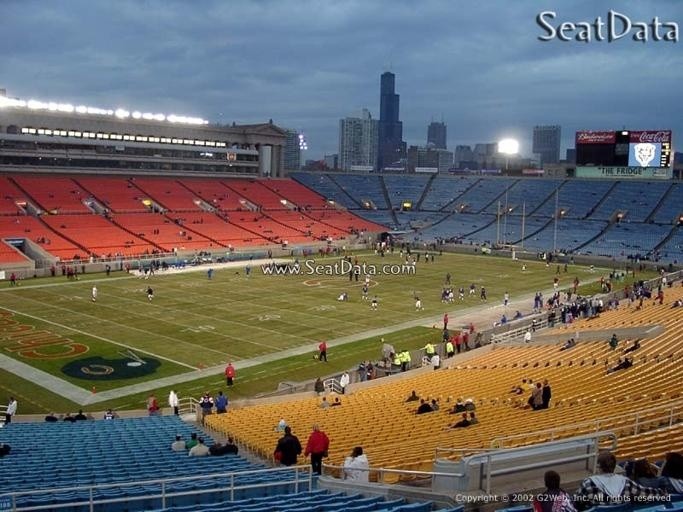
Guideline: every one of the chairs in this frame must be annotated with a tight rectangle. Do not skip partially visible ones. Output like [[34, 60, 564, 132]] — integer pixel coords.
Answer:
[[0, 169, 682, 512]]
